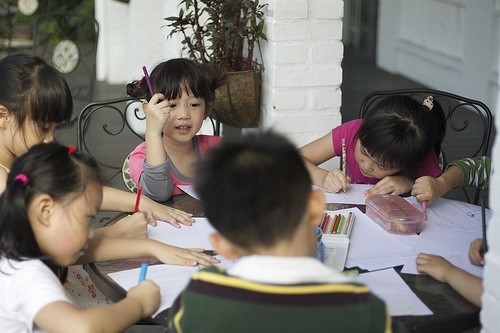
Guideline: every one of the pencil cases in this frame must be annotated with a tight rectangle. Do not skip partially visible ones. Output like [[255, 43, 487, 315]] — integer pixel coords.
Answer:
[[317, 211, 355, 273], [365, 194, 427, 235]]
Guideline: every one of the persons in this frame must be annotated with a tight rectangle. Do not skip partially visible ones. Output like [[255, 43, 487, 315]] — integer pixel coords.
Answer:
[[416, 236, 489, 333], [0, 54, 196, 229], [295, 95, 446, 197], [125, 58, 227, 203], [412, 154, 496, 204], [0, 142, 221, 333], [170, 130, 391, 333]]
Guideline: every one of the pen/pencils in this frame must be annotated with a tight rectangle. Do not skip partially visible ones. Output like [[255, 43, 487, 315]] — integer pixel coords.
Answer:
[[143, 66, 154, 97], [138, 263, 147, 281], [481, 197, 488, 255], [134, 187, 142, 212], [422, 200, 427, 221], [341, 137, 346, 193], [317, 211, 352, 234]]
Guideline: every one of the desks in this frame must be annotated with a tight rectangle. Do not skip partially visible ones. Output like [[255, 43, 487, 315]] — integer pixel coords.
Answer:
[[81, 181, 485, 333]]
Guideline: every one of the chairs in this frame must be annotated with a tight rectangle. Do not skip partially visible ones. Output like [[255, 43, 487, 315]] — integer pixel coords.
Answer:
[[0, 0, 500, 333]]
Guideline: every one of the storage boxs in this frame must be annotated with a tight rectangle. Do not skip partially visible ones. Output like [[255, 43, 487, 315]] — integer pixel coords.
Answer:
[[366, 192, 424, 235]]
